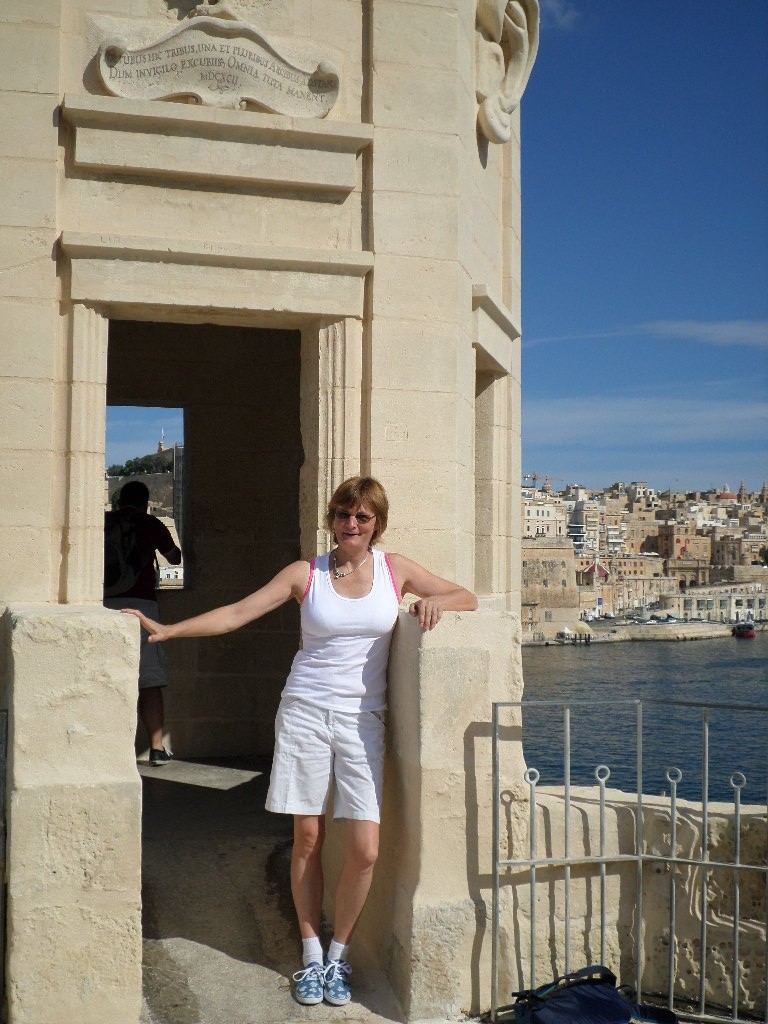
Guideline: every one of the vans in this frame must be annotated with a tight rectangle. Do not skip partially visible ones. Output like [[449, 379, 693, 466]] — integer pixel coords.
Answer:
[[667, 618, 676, 624]]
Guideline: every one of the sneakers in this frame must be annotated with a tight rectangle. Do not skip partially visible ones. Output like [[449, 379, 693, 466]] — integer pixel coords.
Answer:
[[150, 746, 170, 765]]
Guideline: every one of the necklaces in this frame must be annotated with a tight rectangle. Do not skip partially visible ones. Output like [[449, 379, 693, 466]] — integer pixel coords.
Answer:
[[334, 547, 369, 579]]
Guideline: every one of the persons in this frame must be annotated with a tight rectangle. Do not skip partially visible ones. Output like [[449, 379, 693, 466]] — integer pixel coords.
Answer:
[[121, 476, 478, 1004], [104, 482, 182, 764]]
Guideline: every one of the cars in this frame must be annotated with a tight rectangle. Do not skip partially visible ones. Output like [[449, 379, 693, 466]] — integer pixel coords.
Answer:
[[613, 620, 631, 626], [646, 620, 657, 625]]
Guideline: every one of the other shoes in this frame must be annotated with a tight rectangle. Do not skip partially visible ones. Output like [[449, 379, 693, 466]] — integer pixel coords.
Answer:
[[324, 960, 351, 1005], [296, 962, 323, 1005]]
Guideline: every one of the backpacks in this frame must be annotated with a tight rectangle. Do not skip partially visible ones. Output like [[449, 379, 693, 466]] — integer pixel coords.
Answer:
[[511, 966, 679, 1023]]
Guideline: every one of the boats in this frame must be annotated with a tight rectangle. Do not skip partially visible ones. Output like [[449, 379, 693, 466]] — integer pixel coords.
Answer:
[[733, 611, 757, 638]]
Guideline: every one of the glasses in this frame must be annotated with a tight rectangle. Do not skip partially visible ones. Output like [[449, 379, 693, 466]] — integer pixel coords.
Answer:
[[334, 508, 377, 524]]
